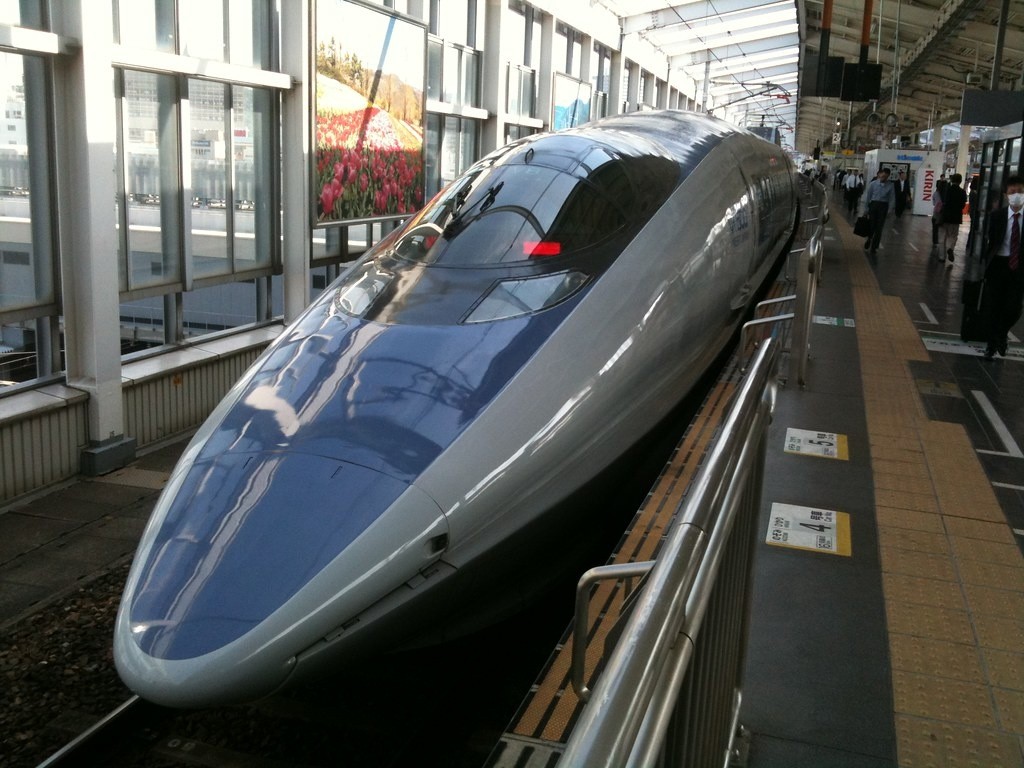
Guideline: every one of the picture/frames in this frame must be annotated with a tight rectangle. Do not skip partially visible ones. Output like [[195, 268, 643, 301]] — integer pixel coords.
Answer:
[[308, 0, 429, 229], [552, 70, 592, 131]]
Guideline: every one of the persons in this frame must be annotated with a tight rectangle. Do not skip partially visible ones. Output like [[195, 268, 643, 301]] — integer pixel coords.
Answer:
[[864, 168, 895, 252], [984, 174, 1024, 357], [804, 167, 912, 218], [932, 173, 978, 263]]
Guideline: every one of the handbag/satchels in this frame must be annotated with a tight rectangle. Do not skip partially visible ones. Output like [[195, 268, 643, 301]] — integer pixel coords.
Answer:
[[960, 277, 985, 341], [853, 213, 870, 237], [934, 199, 942, 213]]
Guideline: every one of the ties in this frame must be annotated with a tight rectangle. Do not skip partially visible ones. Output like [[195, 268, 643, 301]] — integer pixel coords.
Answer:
[[854, 175, 856, 188], [1009, 213, 1021, 270]]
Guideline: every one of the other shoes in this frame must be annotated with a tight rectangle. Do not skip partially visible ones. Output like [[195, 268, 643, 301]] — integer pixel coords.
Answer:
[[947, 249, 953, 262], [871, 247, 876, 253], [939, 258, 945, 263], [984, 338, 998, 358], [996, 334, 1008, 357], [864, 240, 871, 249]]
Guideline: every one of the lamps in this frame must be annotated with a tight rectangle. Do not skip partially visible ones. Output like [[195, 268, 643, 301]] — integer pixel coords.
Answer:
[[965, 48, 983, 85]]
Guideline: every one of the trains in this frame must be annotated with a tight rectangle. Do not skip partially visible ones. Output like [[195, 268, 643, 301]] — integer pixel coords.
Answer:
[[109, 106, 801, 713]]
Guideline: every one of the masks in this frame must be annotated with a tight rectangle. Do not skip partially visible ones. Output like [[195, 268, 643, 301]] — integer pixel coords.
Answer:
[[1006, 193, 1024, 206]]
